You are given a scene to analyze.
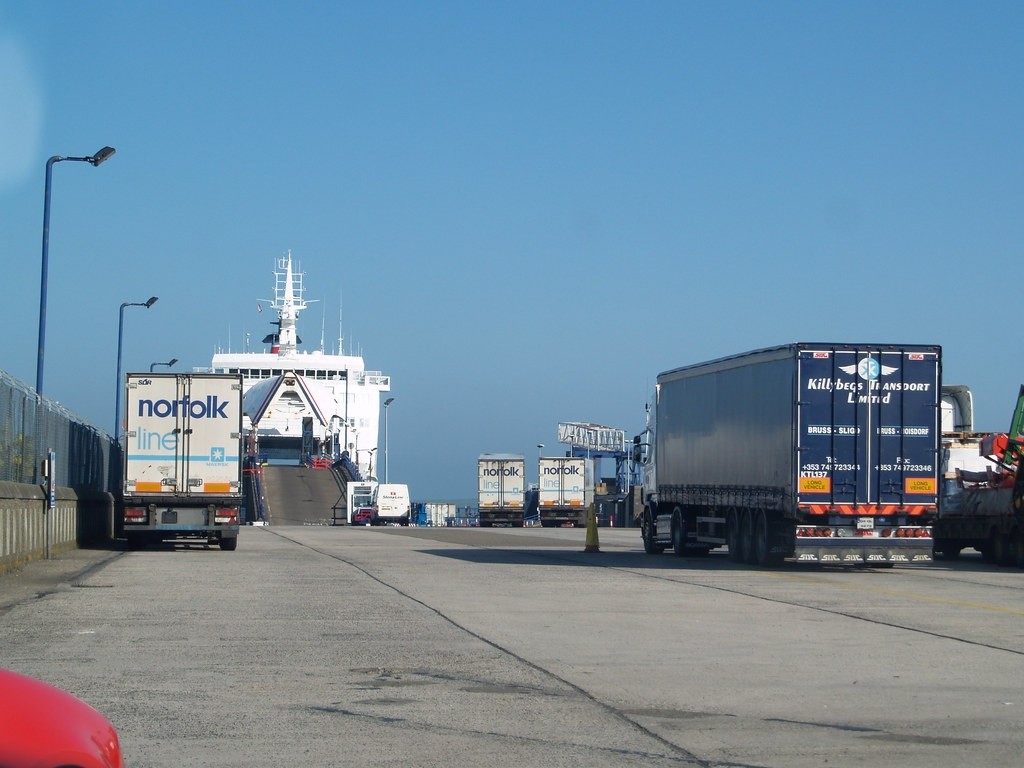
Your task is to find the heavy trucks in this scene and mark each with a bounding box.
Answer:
[933,384,1024,567]
[632,341,942,566]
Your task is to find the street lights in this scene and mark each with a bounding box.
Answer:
[150,358,178,372]
[114,296,159,444]
[384,397,394,483]
[35,146,116,485]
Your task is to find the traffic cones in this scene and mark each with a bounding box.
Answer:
[584,502,600,553]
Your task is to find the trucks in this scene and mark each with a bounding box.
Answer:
[477,452,525,527]
[373,483,411,526]
[538,457,594,527]
[347,482,379,525]
[122,372,244,550]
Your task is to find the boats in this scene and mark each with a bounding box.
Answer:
[210,248,392,482]
